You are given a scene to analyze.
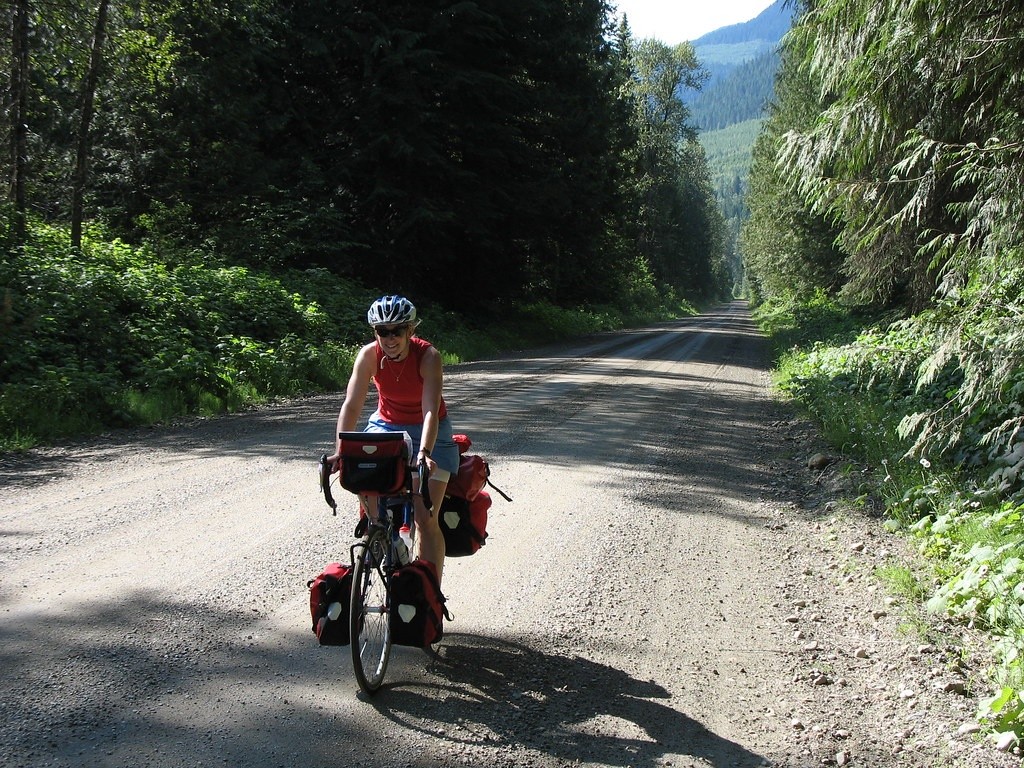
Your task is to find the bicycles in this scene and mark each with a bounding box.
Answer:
[318,433,472,698]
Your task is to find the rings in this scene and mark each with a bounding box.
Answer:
[432,473,435,475]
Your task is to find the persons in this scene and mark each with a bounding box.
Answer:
[583,299,592,314]
[319,296,460,592]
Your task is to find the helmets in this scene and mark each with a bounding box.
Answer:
[368,294,416,327]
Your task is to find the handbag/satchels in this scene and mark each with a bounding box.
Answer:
[446,455,490,502]
[439,491,494,558]
[338,430,412,498]
[388,559,454,647]
[309,562,364,647]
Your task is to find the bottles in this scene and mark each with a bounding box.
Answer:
[400,523,412,563]
[393,531,408,565]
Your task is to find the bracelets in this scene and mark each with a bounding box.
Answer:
[419,446,430,457]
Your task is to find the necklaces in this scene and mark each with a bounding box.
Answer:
[385,354,408,382]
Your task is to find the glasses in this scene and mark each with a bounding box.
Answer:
[375,324,408,338]
